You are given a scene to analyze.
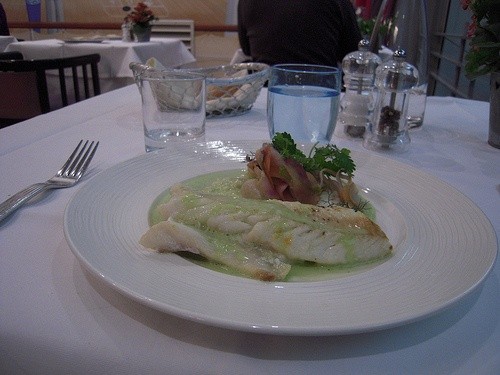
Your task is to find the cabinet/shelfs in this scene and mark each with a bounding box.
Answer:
[151,19,194,56]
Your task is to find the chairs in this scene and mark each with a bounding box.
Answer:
[0,52,101,119]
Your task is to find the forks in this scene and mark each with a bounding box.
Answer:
[0,139,100,221]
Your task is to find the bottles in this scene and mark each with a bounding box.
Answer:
[121,25,130,41]
[372,0,428,129]
[364,49,420,155]
[340,39,382,138]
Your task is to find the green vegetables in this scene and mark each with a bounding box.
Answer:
[242,132,356,177]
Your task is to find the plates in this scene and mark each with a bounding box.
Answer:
[62,137,498,335]
[61,39,103,43]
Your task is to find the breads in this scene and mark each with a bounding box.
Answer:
[193,69,248,100]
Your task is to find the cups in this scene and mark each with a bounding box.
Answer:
[135,70,207,152]
[267,64,342,146]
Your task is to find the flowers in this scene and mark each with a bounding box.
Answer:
[129,2,158,25]
[462,0,500,80]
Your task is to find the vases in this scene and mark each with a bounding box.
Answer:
[488,70,500,149]
[134,25,152,42]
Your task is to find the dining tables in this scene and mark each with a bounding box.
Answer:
[0,82,500,375]
[4,38,196,115]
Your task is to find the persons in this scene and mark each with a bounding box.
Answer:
[237,0,360,92]
